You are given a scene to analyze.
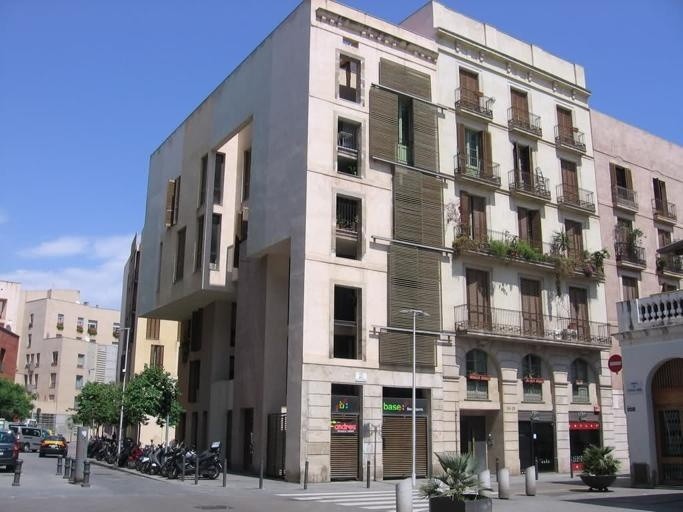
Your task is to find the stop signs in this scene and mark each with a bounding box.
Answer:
[608,355,623,373]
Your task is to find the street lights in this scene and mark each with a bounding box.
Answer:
[116,328,130,467]
[399,307,430,487]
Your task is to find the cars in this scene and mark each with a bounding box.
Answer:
[0,418,70,473]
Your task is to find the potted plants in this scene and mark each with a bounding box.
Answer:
[578,441,617,492]
[419,451,495,512]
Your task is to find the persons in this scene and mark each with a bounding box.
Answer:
[112,431,116,440]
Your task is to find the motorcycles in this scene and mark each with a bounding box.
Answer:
[87,432,223,479]
